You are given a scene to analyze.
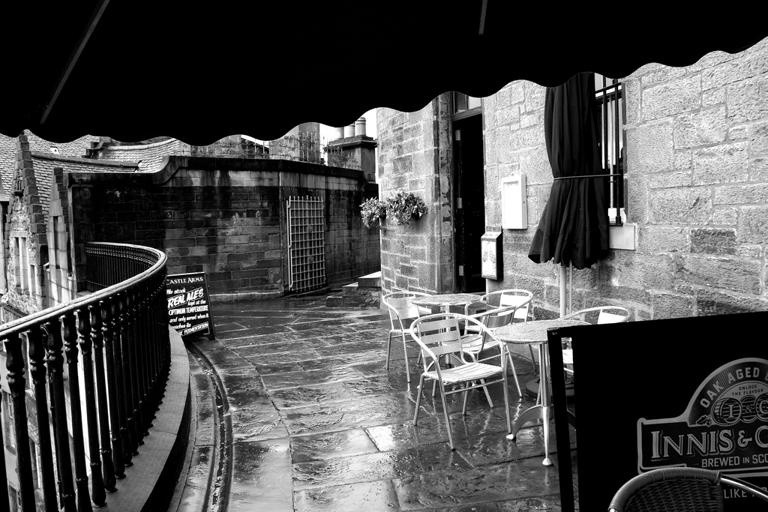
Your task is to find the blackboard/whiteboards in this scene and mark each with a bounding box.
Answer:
[166,271,213,336]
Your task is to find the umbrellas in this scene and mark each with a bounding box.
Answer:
[528,70,610,317]
[0,0,768,145]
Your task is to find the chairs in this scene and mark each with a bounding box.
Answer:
[381,285,633,452]
[607,463,768,512]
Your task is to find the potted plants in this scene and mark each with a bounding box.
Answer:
[357,187,428,231]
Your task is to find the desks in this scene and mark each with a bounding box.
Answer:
[497,316,592,468]
